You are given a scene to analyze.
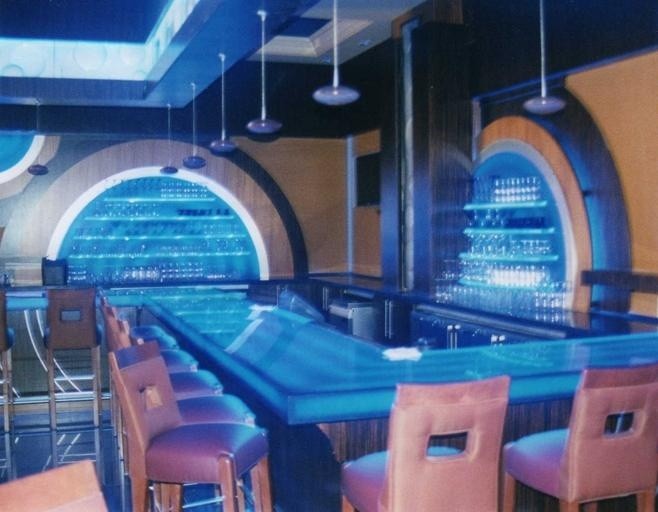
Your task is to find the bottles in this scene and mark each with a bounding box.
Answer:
[432,174,568,315]
[64,176,249,286]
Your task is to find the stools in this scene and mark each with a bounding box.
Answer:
[40,284,104,430]
[338,378,509,509]
[0,287,21,435]
[98,294,275,512]
[503,368,658,509]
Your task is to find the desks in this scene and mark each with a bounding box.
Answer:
[292,268,657,346]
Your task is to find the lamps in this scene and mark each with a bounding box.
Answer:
[244,12,285,145]
[520,1,565,121]
[182,80,207,173]
[161,102,178,174]
[207,50,240,158]
[28,98,49,176]
[309,3,359,109]
